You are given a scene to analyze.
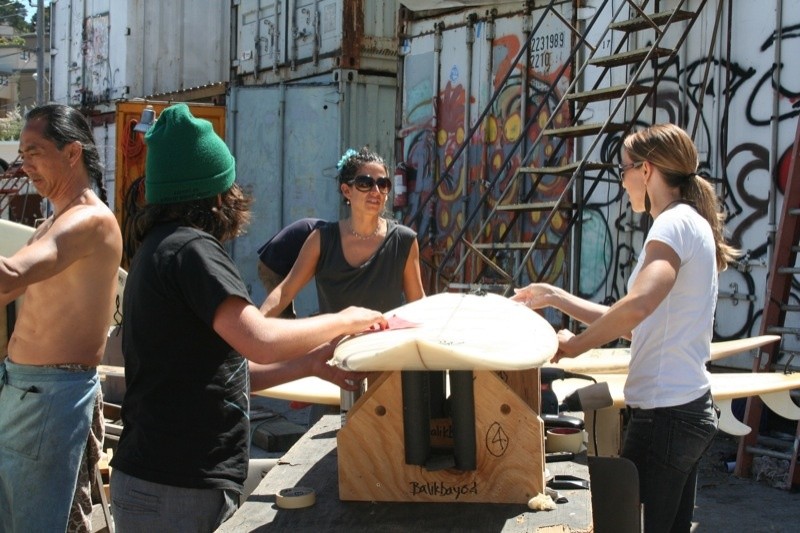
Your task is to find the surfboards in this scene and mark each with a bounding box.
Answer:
[333,291,560,372]
[0,219,129,325]
[249,333,800,407]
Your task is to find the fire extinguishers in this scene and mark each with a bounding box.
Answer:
[393,162,408,207]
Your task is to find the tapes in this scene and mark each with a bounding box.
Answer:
[275,487,316,509]
[547,428,583,453]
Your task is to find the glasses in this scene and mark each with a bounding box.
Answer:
[346,174,392,194]
[617,160,644,181]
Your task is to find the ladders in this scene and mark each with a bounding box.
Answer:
[734,119,800,491]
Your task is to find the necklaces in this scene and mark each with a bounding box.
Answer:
[348,215,382,241]
[660,198,682,214]
[47,188,88,226]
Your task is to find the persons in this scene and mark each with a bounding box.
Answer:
[106,105,390,533]
[509,124,743,533]
[257,218,329,319]
[257,148,428,428]
[0,105,123,533]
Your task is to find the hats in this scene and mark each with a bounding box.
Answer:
[144,104,237,201]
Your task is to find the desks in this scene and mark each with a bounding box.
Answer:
[215,415,595,533]
[546,334,780,370]
[248,373,800,455]
